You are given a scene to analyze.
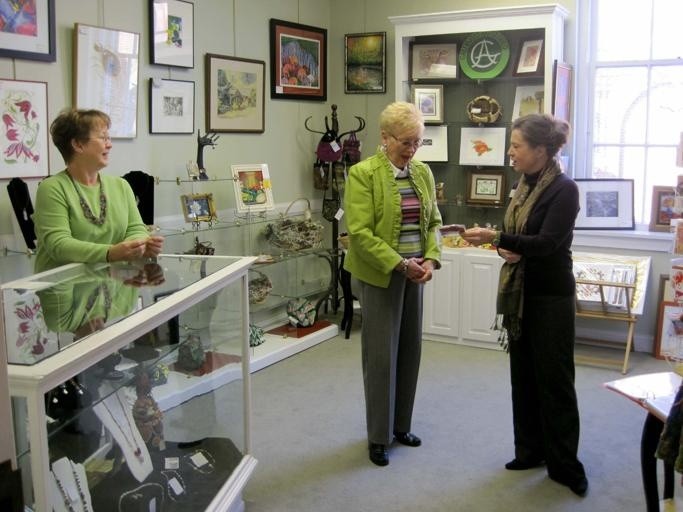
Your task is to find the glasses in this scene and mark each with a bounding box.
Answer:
[77,130,112,141]
[385,128,422,148]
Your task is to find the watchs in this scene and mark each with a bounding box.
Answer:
[395,258,409,274]
[492,231,502,249]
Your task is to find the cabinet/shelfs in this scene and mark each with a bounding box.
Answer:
[3,250,260,511]
[45,178,339,412]
[380,5,572,352]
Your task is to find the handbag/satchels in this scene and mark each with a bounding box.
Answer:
[343,131,361,164]
[266,198,322,256]
[196,242,214,256]
[178,339,202,369]
[318,130,341,161]
[285,298,319,326]
[322,167,340,221]
[313,157,330,189]
[249,268,272,304]
[252,326,262,346]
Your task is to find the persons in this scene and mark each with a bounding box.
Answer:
[34,260,164,341]
[460,112,589,497]
[32,108,164,271]
[346,100,443,465]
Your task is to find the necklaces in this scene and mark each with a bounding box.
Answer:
[119,482,165,512]
[160,468,187,505]
[66,168,107,225]
[182,450,217,476]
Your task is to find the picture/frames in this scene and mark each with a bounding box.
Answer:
[648,184,680,234]
[268,17,328,105]
[0,79,50,181]
[150,0,194,70]
[72,23,139,140]
[404,82,443,121]
[204,53,266,136]
[656,299,683,361]
[406,40,460,82]
[179,193,218,225]
[570,178,635,232]
[511,84,544,122]
[148,76,196,137]
[463,174,506,206]
[0,1,57,65]
[654,274,683,356]
[229,164,276,215]
[344,31,386,96]
[513,36,542,74]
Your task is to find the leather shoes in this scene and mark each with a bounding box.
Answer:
[368,442,389,465]
[569,478,587,494]
[394,431,420,447]
[506,458,525,470]
